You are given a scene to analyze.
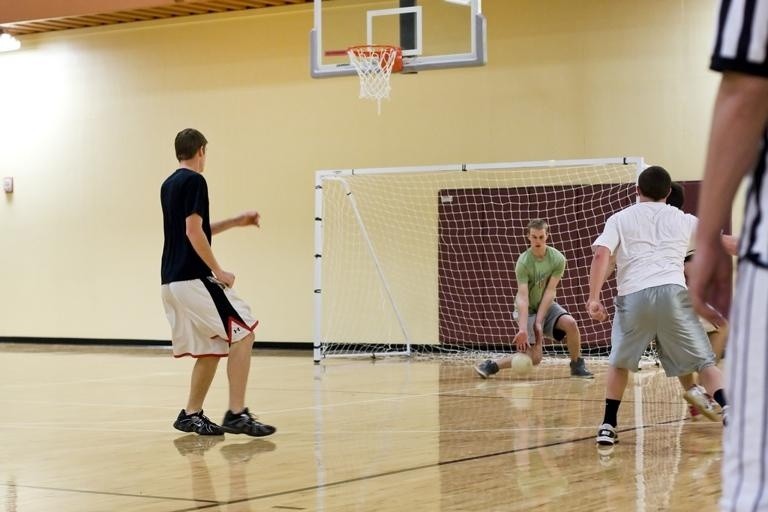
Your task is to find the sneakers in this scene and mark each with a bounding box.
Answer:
[174,409,224,435]
[595,423,619,445]
[221,407,275,436]
[570,357,594,379]
[685,384,730,426]
[474,360,499,379]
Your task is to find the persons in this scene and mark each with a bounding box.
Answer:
[509,380,572,511]
[652,182,730,424]
[156,128,275,438]
[583,164,730,448]
[170,433,276,512]
[684,0,768,509]
[593,439,690,512]
[474,217,597,380]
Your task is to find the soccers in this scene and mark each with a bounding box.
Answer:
[511,354,533,376]
